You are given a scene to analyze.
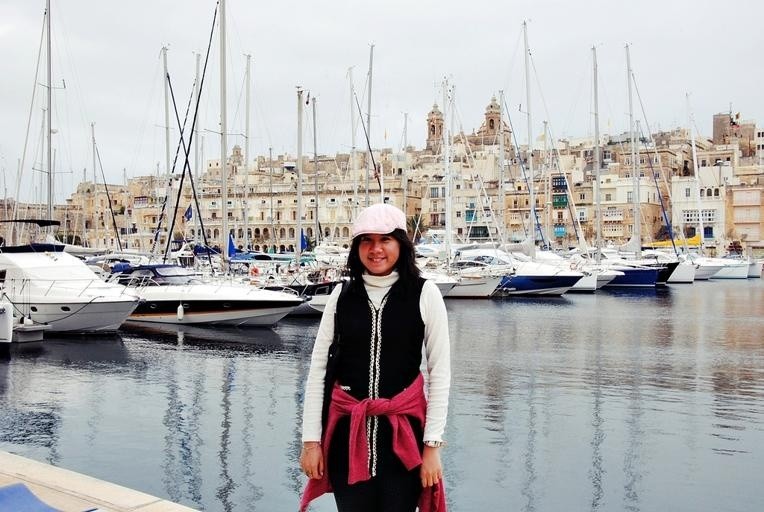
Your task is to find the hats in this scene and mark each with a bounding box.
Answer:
[347,200,409,242]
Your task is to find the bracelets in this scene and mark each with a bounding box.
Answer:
[303,444,320,450]
[423,441,443,448]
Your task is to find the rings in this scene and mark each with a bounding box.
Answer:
[305,471,311,477]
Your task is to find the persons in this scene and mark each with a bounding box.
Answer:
[300,204,451,512]
